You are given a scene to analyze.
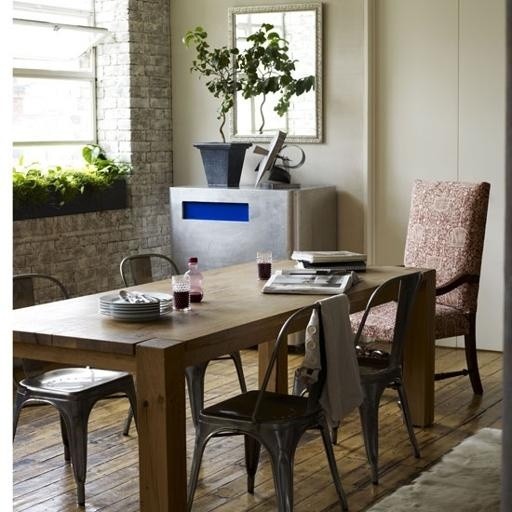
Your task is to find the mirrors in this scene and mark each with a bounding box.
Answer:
[228,2,323,145]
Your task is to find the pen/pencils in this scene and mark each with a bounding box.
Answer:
[290,269,347,275]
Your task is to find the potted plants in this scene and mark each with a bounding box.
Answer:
[182,26,245,187]
[14,142,127,218]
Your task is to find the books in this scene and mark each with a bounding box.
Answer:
[253,247,369,296]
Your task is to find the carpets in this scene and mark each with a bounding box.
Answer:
[365,429,501,510]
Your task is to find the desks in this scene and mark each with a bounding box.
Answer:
[11,259,437,512]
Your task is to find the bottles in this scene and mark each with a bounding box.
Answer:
[184,254,203,303]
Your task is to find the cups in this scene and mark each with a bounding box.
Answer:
[257,250,272,281]
[171,273,190,313]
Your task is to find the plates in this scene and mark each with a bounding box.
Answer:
[98,290,174,324]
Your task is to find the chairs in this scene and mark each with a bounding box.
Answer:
[11,272,138,506]
[349,179,490,410]
[290,271,423,484]
[119,253,248,443]
[185,292,350,512]
